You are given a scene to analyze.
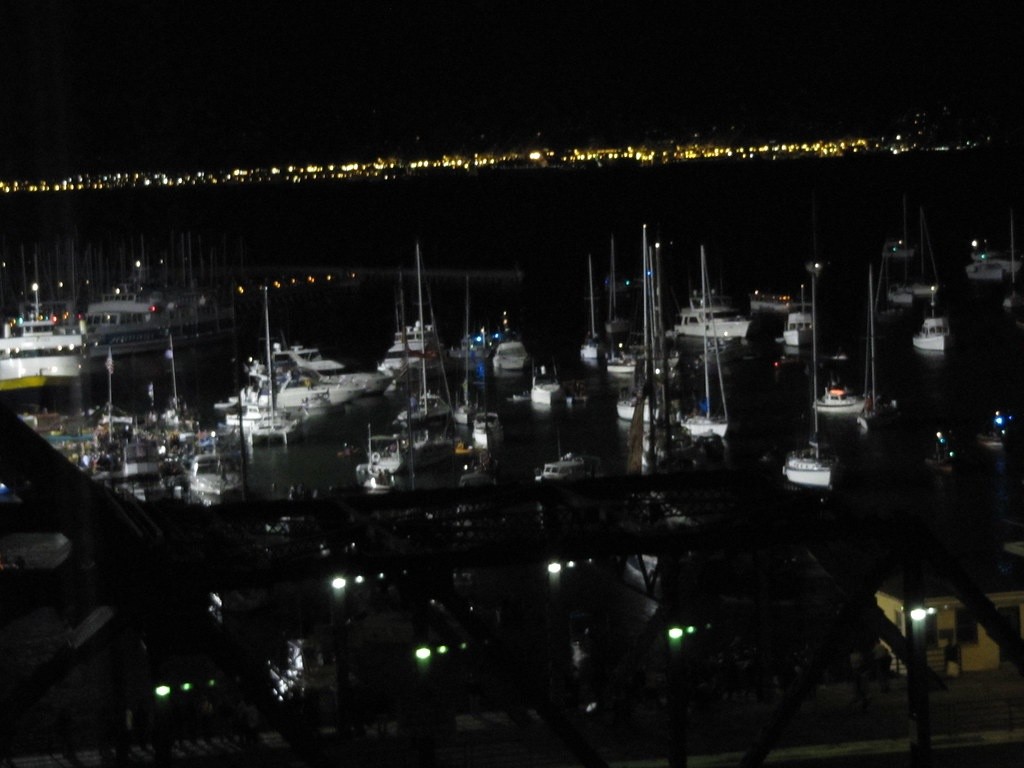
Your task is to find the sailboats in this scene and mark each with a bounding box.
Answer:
[0,205,1024,507]
